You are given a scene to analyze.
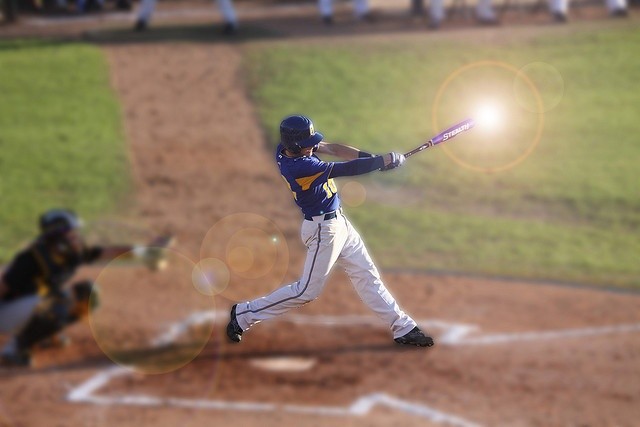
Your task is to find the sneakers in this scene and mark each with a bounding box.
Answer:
[394,326,434,348]
[227,304,243,342]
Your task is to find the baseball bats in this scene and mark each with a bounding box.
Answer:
[404,116,475,158]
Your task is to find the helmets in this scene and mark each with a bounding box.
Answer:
[40,210,83,274]
[280,115,324,154]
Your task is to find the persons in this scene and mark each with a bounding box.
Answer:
[226,114,434,347]
[426,1,501,27]
[315,1,376,21]
[131,2,243,32]
[549,1,632,20]
[1,207,149,365]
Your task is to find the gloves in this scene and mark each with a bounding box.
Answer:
[389,152,406,167]
[375,155,396,170]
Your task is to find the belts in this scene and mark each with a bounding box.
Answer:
[305,205,342,221]
